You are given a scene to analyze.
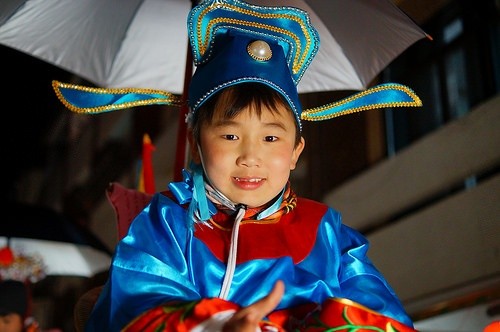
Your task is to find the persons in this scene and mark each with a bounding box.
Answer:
[51,0,422,332]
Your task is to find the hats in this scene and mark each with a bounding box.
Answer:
[52,0,422,133]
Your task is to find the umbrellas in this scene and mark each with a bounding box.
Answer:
[0,0,433,182]
